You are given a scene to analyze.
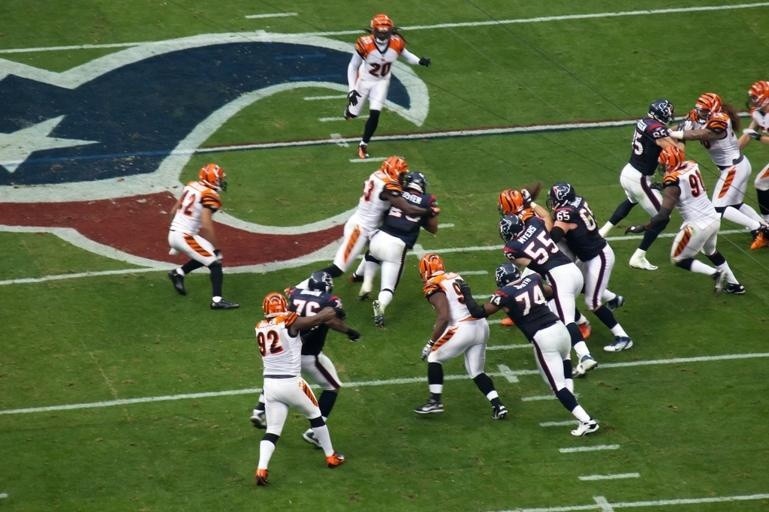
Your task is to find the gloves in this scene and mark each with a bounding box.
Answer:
[625,225,644,235]
[420,340,434,361]
[348,91,361,105]
[334,307,346,320]
[419,57,430,67]
[456,280,469,289]
[650,182,663,191]
[346,329,360,341]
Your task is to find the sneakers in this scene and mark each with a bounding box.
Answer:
[572,295,634,379]
[491,405,509,420]
[629,257,659,270]
[413,399,445,414]
[168,269,186,295]
[349,272,384,328]
[500,317,513,326]
[302,428,322,448]
[344,109,351,121]
[326,451,345,468]
[570,418,600,437]
[751,228,769,250]
[358,145,369,159]
[711,269,746,295]
[256,469,269,486]
[210,299,240,310]
[250,409,267,429]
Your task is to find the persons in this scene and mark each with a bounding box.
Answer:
[167,163,239,310]
[598,82,769,294]
[284,156,439,329]
[415,253,508,419]
[456,181,634,435]
[342,14,431,158]
[249,272,360,486]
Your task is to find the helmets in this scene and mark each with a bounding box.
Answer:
[382,155,426,195]
[647,80,769,125]
[199,163,227,192]
[371,14,394,44]
[419,253,445,283]
[309,271,335,293]
[262,292,289,319]
[494,262,522,286]
[656,145,686,175]
[495,182,524,243]
[547,183,576,210]
